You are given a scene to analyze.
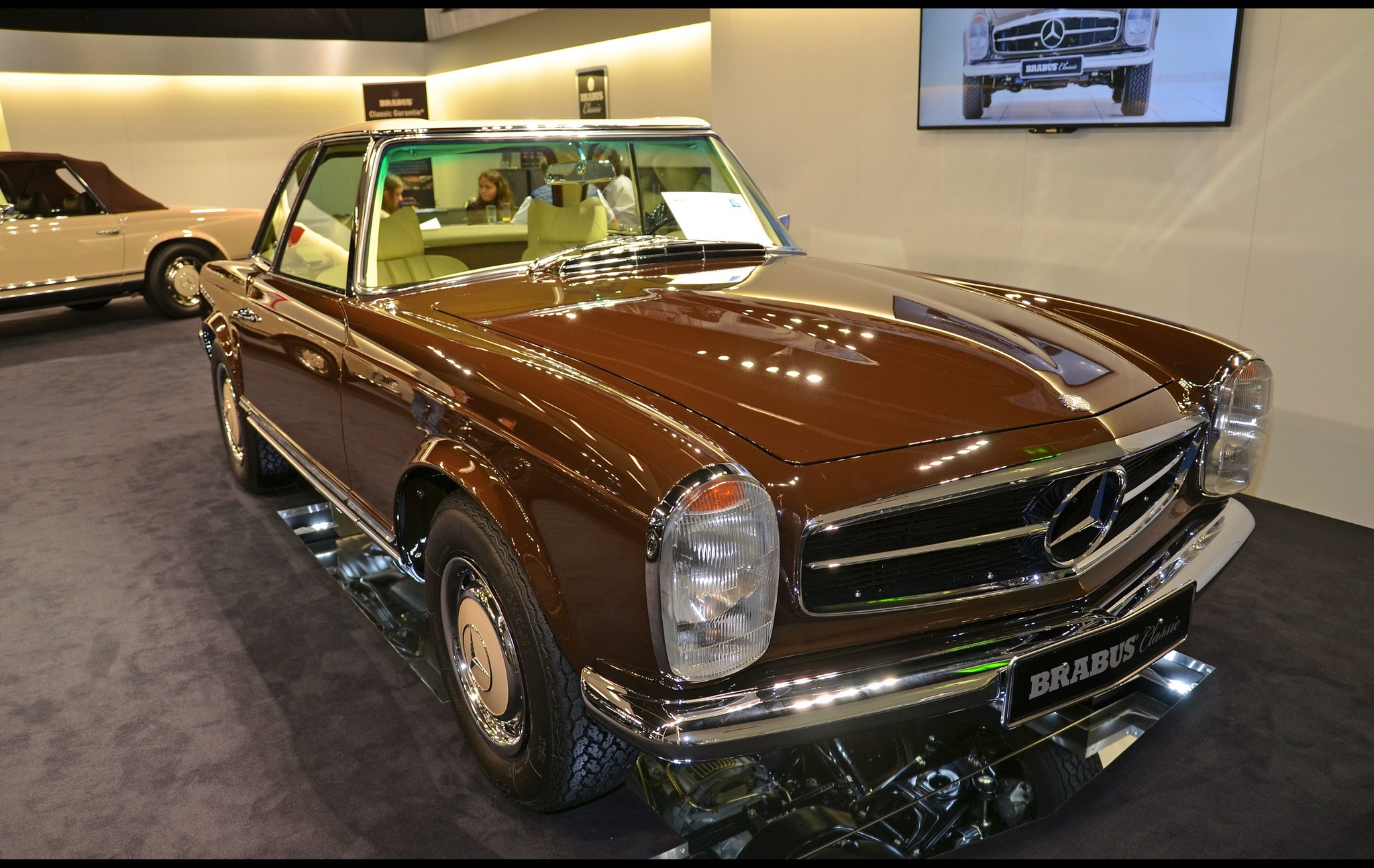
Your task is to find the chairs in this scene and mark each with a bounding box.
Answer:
[377,206,469,287]
[62,192,95,215]
[17,194,50,217]
[521,196,607,263]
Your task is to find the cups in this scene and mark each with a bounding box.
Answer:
[501,202,511,222]
[486,205,497,224]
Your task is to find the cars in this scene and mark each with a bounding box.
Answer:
[200,114,1272,858]
[0,151,268,320]
[963,9,1161,120]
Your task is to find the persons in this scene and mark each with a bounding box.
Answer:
[591,144,644,231]
[467,169,516,224]
[508,153,618,230]
[339,171,404,233]
